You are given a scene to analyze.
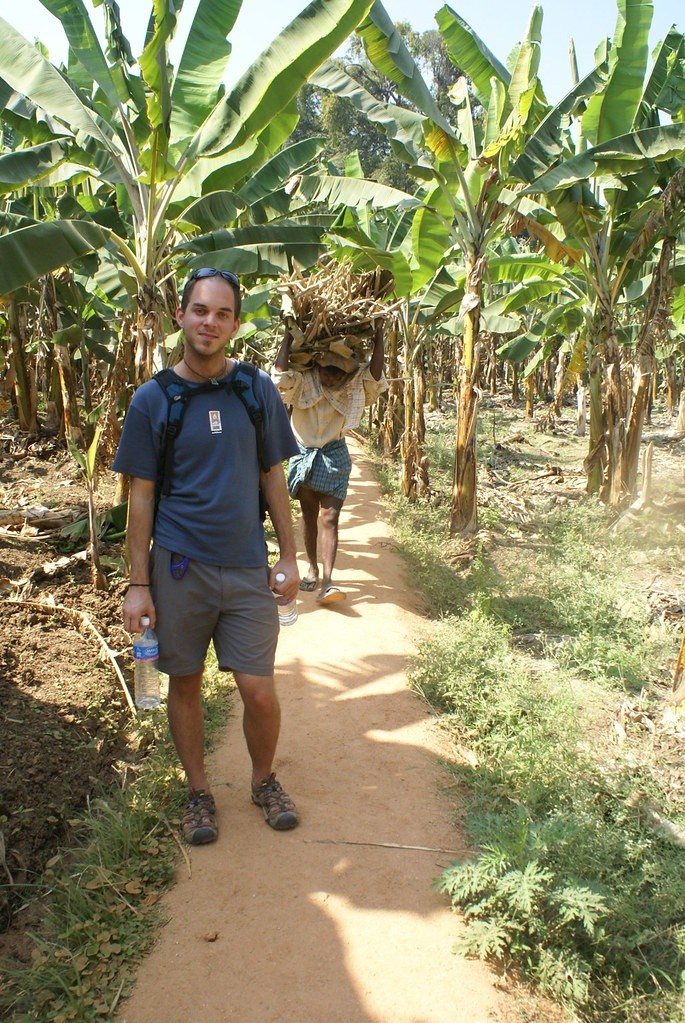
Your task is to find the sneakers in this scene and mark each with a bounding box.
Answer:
[180,786,218,844]
[251,772,300,831]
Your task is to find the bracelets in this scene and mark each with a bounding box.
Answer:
[128,583,150,587]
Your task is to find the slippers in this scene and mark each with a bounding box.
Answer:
[298,575,319,591]
[316,585,346,605]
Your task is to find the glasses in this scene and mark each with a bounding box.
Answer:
[191,267,240,290]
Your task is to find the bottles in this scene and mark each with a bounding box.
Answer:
[133,616,161,709]
[272,573,298,626]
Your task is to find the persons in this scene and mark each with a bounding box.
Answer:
[109,268,301,845]
[270,309,391,605]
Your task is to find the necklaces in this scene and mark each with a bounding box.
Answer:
[183,357,227,385]
